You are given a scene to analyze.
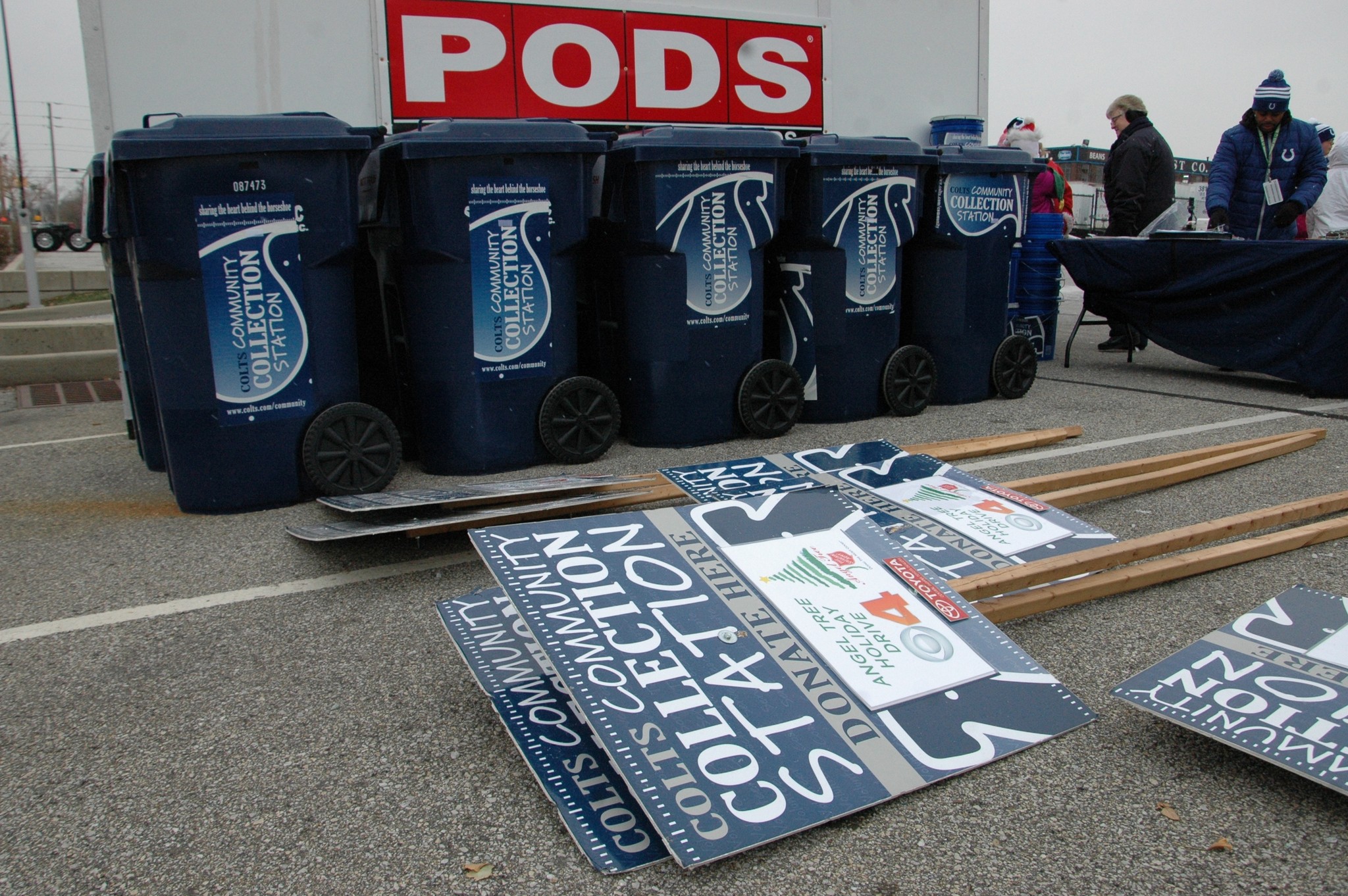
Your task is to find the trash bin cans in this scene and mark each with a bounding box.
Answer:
[84,110,1038,514]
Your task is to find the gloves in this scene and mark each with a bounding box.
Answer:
[1270,202,1302,226]
[1207,209,1230,232]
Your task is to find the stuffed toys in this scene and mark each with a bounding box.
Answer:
[996,115,1074,236]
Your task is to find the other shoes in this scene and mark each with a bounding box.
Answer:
[1098,330,1146,353]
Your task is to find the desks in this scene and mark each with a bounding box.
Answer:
[1058,228,1348,401]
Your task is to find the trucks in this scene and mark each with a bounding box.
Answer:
[1067,180,1210,239]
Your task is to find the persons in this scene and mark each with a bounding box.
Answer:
[1204,68,1330,241]
[1006,126,1065,214]
[1097,94,1176,352]
[1304,131,1348,239]
[1292,118,1335,240]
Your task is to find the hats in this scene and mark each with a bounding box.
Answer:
[1309,118,1335,144]
[1252,70,1291,113]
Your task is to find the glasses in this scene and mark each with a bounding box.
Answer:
[1110,114,1122,125]
